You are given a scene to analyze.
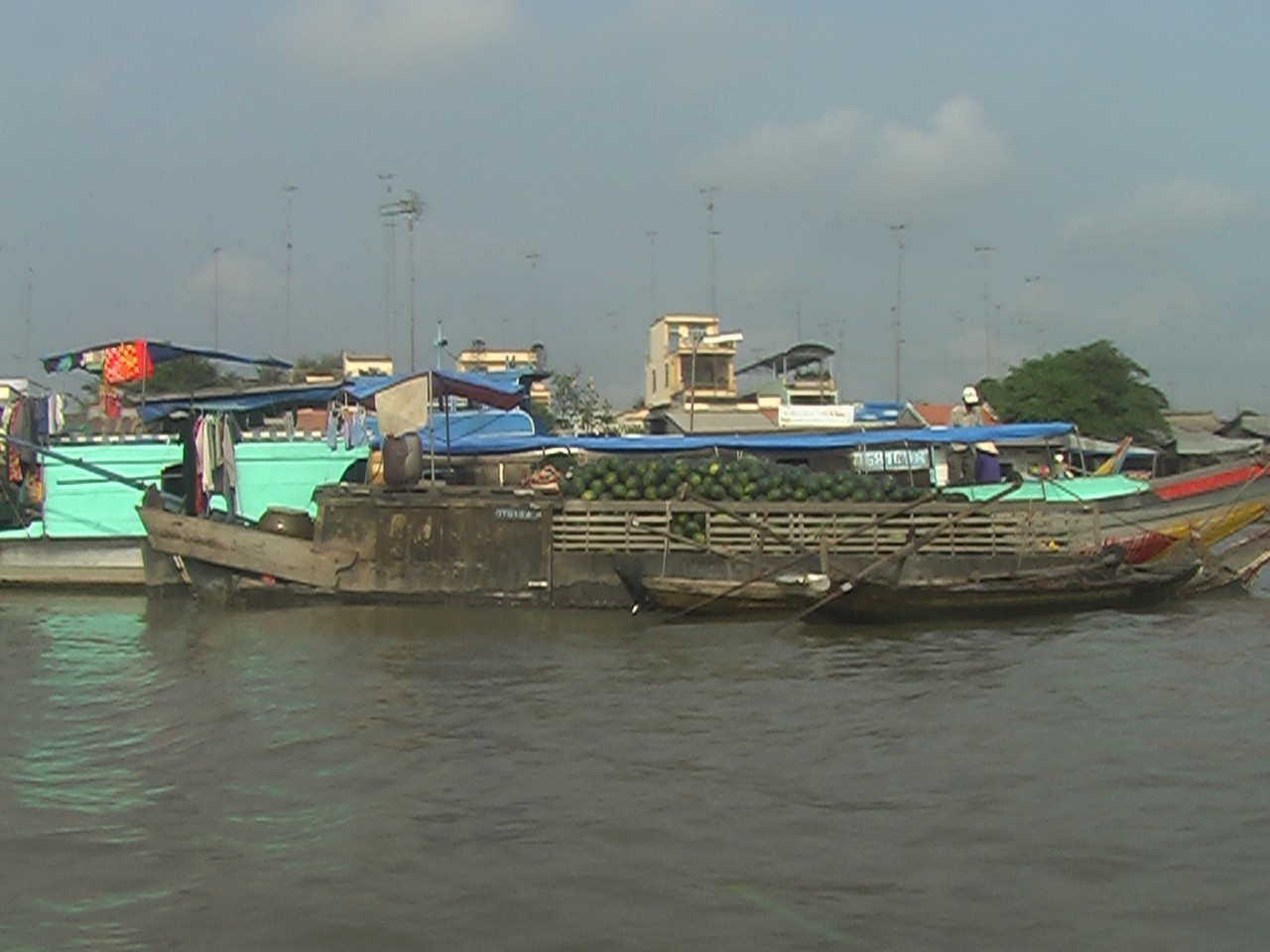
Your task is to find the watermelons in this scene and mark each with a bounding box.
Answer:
[569,453,967,553]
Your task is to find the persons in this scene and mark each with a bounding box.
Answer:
[947,386,979,485]
[975,441,1000,483]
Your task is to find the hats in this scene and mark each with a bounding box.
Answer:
[963,387,978,404]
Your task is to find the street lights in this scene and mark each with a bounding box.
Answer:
[381,189,422,374]
[890,224,905,419]
[213,246,223,350]
[286,184,295,361]
[975,245,992,375]
[380,171,394,359]
[526,251,541,347]
[701,185,722,314]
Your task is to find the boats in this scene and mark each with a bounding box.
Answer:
[0,340,1270,624]
